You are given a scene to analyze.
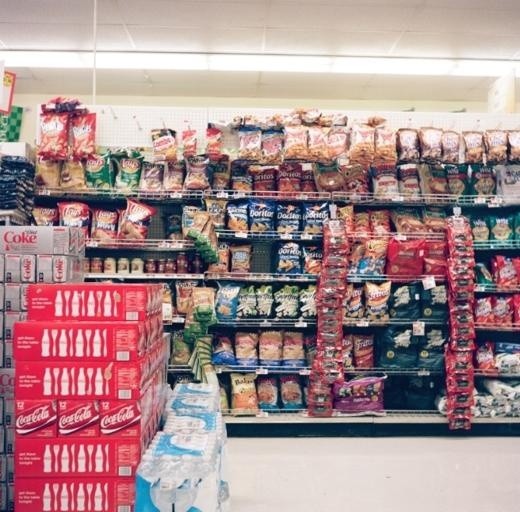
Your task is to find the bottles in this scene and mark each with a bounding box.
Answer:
[99,402,144,436]
[41,328,50,357]
[43,483,51,511]
[57,402,100,434]
[72,291,79,317]
[43,445,52,473]
[61,368,70,395]
[61,445,69,471]
[94,483,102,511]
[95,368,103,395]
[61,483,68,511]
[16,405,58,433]
[78,444,86,472]
[78,368,86,396]
[75,328,84,357]
[88,291,95,316]
[77,483,84,511]
[59,328,68,357]
[43,368,52,395]
[93,328,102,357]
[54,291,63,316]
[104,291,112,317]
[95,445,103,472]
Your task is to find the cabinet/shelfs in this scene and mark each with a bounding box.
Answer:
[32,186,520,438]
